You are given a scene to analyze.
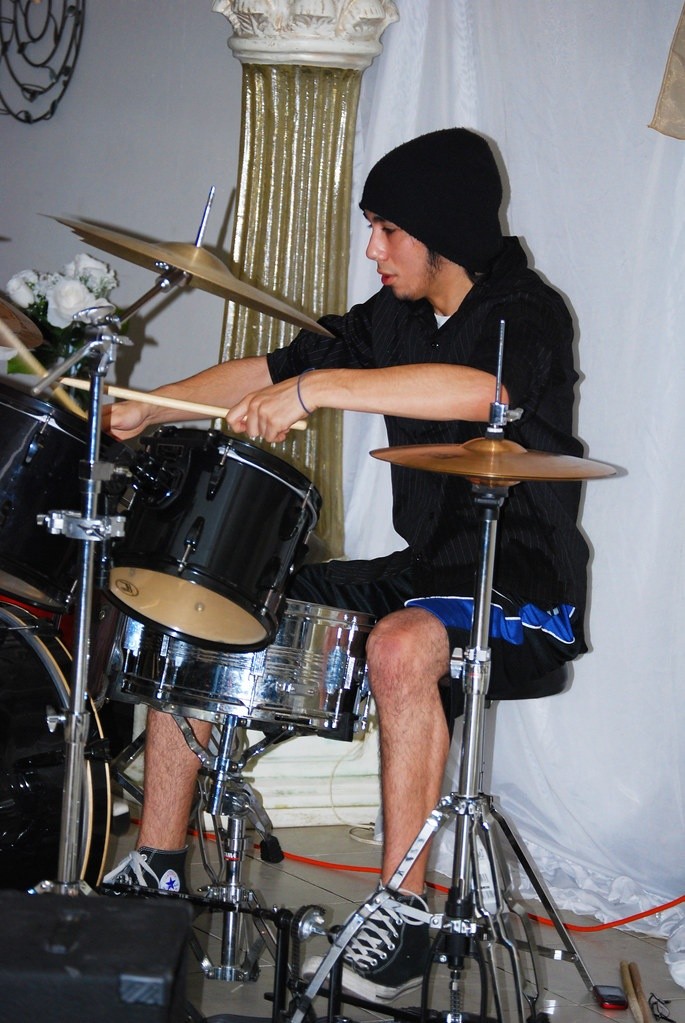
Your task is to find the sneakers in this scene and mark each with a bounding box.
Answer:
[302,894,440,1005]
[94,850,191,899]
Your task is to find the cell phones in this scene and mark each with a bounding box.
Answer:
[593,985,628,1009]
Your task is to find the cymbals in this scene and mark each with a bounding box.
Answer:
[0,295,45,349]
[34,211,337,341]
[368,437,619,487]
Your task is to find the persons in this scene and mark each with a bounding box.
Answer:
[101,128,592,1004]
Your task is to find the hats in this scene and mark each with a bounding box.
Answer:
[360,126,507,268]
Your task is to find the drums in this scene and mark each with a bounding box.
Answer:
[123,594,379,745]
[100,424,324,655]
[0,600,112,899]
[0,383,135,615]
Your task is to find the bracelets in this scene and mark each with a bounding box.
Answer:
[296,367,315,415]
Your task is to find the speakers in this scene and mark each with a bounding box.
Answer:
[0,890,205,1023]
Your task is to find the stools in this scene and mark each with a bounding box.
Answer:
[345,637,575,960]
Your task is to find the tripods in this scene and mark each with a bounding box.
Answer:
[195,484,595,1023]
